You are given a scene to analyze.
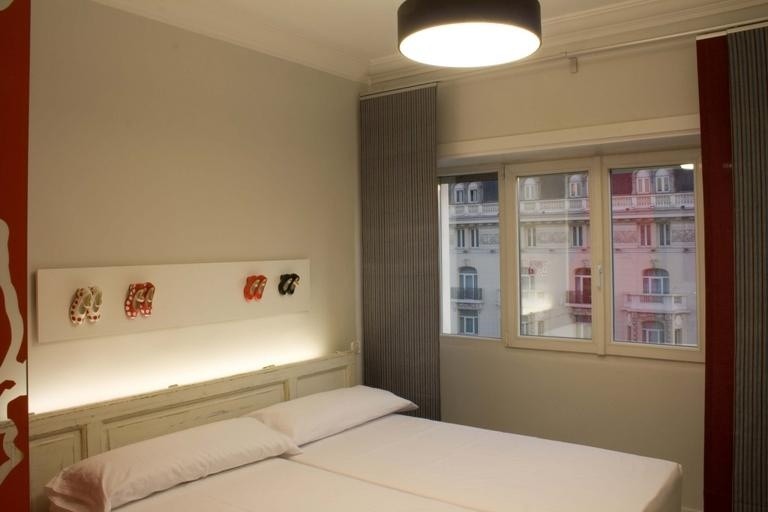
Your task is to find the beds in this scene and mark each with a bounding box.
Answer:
[29,351,683,512]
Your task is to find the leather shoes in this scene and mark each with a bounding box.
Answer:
[69,287,93,326]
[244,275,260,302]
[254,274,267,301]
[140,282,156,319]
[287,273,301,295]
[125,282,145,320]
[86,285,104,323]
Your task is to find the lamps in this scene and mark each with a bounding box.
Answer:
[397,0,541,69]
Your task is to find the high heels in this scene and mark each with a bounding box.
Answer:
[278,273,293,296]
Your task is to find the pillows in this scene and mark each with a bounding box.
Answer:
[242,385,419,446]
[45,416,302,512]
